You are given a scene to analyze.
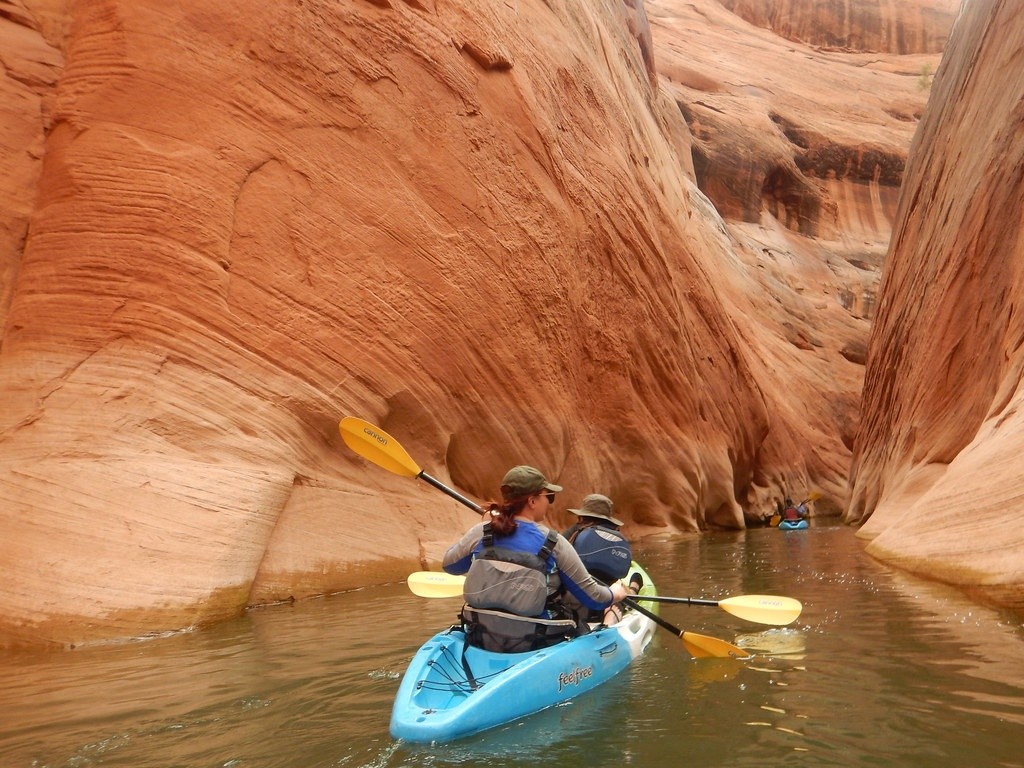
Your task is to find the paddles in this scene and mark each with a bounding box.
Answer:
[337,413,753,662]
[405,569,804,627]
[770,490,823,527]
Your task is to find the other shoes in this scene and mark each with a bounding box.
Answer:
[629,573,643,595]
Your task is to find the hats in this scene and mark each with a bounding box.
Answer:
[501,466,563,499]
[566,494,624,527]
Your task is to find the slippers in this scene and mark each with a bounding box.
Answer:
[602,603,623,623]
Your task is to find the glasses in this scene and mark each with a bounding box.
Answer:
[533,490,555,504]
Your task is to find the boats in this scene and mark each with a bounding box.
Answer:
[779,520,809,530]
[388,559,660,743]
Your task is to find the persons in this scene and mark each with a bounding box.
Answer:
[549,494,643,621]
[772,496,806,522]
[442,465,628,653]
